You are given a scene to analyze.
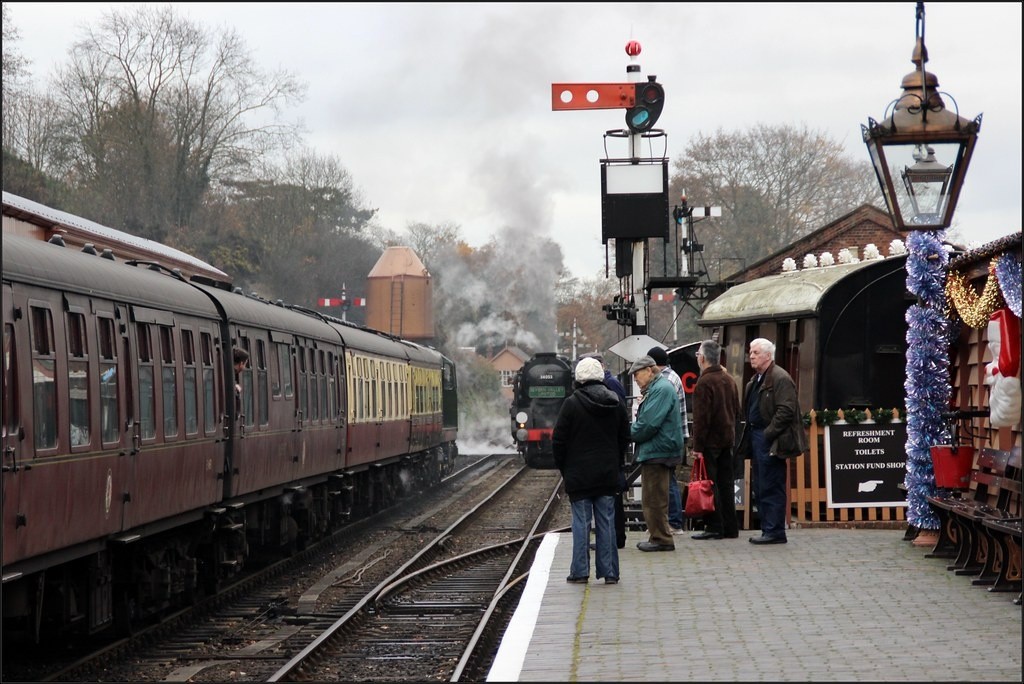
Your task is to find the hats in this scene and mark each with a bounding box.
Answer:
[628,355,656,375]
[580,352,603,363]
[574,357,605,384]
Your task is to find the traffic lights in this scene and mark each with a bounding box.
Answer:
[625,74,665,133]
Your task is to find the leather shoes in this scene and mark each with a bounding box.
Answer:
[567,575,589,583]
[723,532,738,538]
[691,530,723,540]
[605,576,618,584]
[749,536,786,544]
[590,544,597,550]
[636,542,675,551]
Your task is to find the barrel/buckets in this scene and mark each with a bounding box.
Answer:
[930,424,974,490]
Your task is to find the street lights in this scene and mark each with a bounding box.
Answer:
[860,1,984,548]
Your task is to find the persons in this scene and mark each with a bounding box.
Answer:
[692,339,742,539]
[735,337,808,546]
[550,358,632,584]
[233,346,249,395]
[579,352,628,551]
[629,355,684,552]
[636,346,690,535]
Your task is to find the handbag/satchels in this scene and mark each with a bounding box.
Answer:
[684,456,715,517]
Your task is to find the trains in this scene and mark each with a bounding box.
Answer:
[0,189,459,655]
[509,352,580,471]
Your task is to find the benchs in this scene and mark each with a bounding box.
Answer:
[924,447,1010,570]
[954,446,1024,585]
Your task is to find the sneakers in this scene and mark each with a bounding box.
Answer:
[647,524,683,536]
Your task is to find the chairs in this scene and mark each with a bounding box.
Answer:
[982,517,1023,606]
